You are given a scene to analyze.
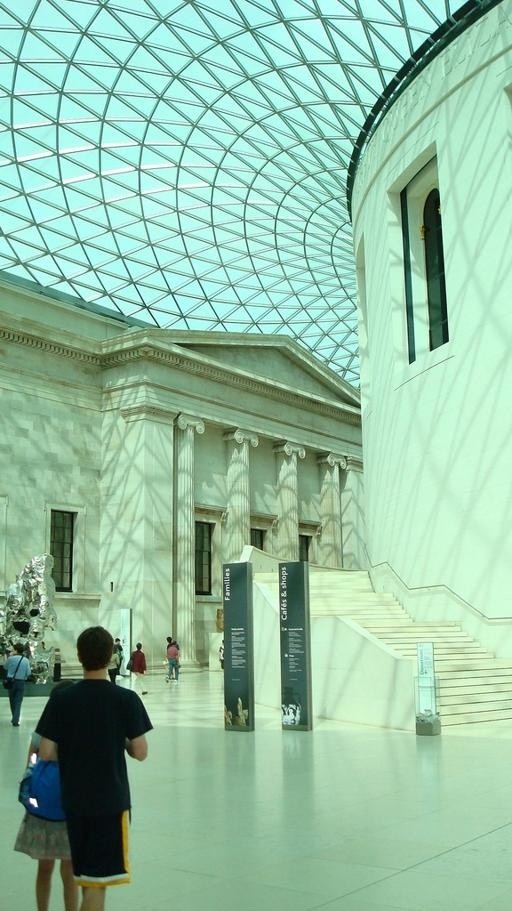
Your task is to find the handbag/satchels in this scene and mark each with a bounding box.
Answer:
[126,659,133,671]
[3,677,15,689]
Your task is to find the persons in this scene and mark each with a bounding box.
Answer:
[3,643,32,726]
[12,679,80,911]
[130,642,149,695]
[166,637,180,681]
[108,637,123,686]
[33,625,153,911]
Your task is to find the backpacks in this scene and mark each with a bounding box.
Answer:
[17,759,69,823]
